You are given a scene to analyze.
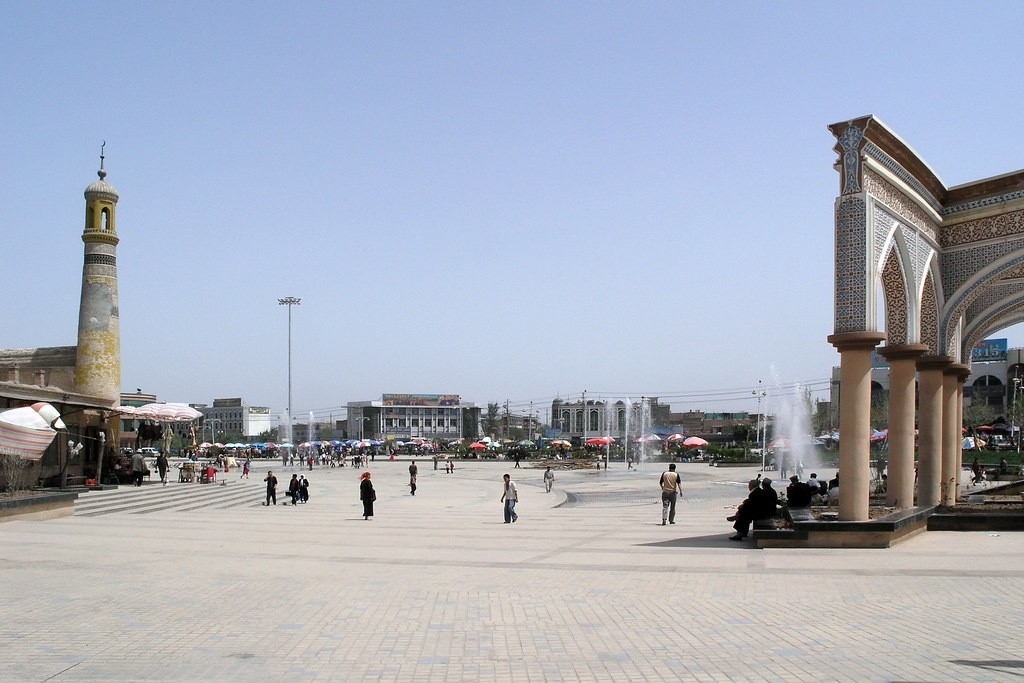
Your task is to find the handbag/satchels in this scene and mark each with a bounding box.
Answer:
[286,490,292,496]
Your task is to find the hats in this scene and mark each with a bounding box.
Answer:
[763,478,772,485]
[137,449,143,452]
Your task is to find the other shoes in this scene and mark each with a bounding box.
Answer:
[513,516,518,522]
[727,516,736,521]
[729,535,742,541]
[504,522,510,523]
[662,521,666,525]
[670,522,675,524]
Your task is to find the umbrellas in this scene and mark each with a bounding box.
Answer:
[636,432,662,444]
[976,424,992,431]
[354,441,370,449]
[329,440,346,448]
[199,441,213,447]
[280,443,294,449]
[211,442,225,449]
[517,438,536,448]
[225,442,235,448]
[668,434,686,444]
[469,442,487,451]
[769,436,792,449]
[1006,425,1020,432]
[369,440,381,446]
[550,439,572,449]
[683,437,709,448]
[234,443,247,448]
[992,423,1008,431]
[299,441,312,448]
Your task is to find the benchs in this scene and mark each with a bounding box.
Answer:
[54,476,86,486]
[108,474,120,485]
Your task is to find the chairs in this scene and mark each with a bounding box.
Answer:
[142,470,150,482]
[183,463,216,484]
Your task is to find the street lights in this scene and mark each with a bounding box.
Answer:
[218,430,223,443]
[202,426,211,443]
[522,409,540,441]
[460,406,470,438]
[356,410,370,439]
[752,380,767,448]
[277,296,302,444]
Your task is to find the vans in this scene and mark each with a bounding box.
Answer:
[142,448,159,453]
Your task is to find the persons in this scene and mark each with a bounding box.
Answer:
[130,431,1024,541]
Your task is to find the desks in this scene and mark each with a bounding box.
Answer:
[179,468,191,482]
[201,470,217,484]
